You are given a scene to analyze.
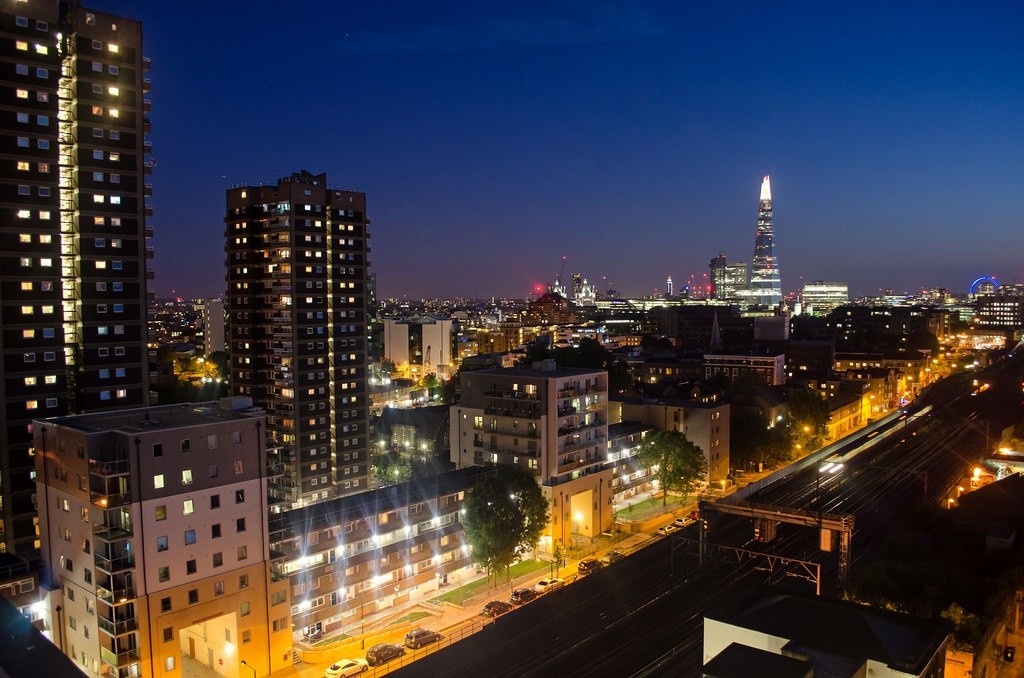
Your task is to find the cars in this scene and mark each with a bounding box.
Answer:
[689,511,699,520]
[482,601,513,618]
[324,658,370,678]
[510,587,539,605]
[657,524,677,536]
[535,577,565,594]
[675,517,693,527]
[602,551,624,564]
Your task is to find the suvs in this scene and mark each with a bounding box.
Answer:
[366,643,404,666]
[578,558,603,574]
[404,628,442,649]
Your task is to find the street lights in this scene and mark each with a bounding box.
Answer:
[436,571,441,605]
[393,586,400,625]
[957,486,964,499]
[947,498,955,510]
[720,480,726,495]
[360,579,381,650]
[575,512,583,554]
[796,445,801,461]
[241,660,256,678]
